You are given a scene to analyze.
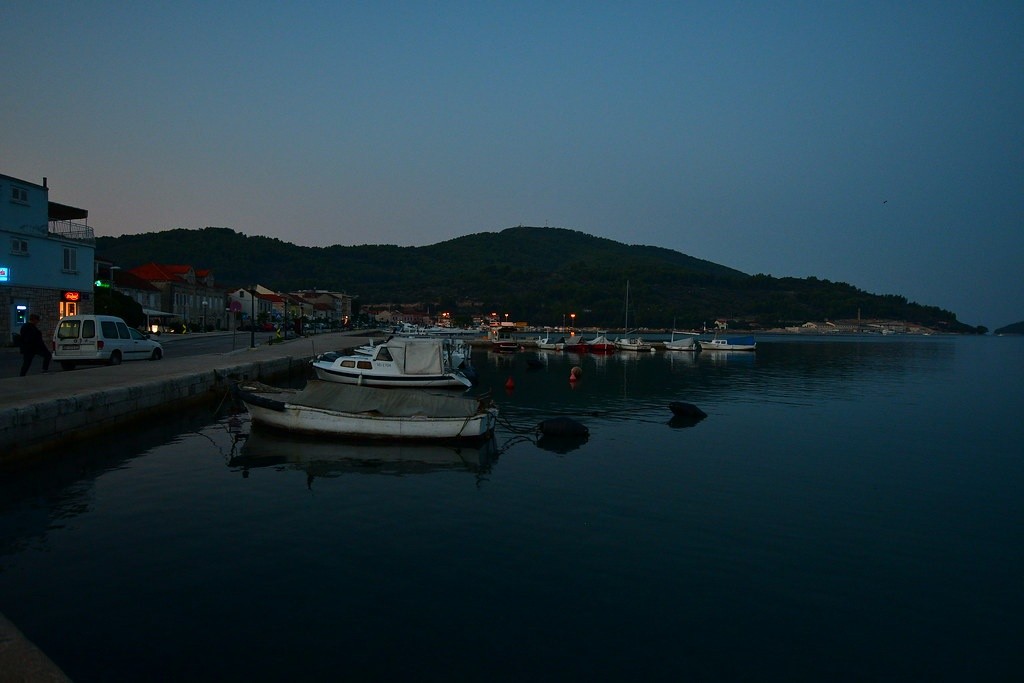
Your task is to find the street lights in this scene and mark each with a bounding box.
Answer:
[312,307,317,335]
[202,301,210,333]
[299,300,305,337]
[280,294,288,340]
[248,282,258,350]
[225,307,230,331]
[330,312,334,333]
[108,266,121,298]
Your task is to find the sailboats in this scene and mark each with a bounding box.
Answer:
[614,279,651,351]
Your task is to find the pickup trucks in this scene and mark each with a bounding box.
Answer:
[50,314,165,371]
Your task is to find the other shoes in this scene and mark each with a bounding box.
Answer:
[42,370,55,375]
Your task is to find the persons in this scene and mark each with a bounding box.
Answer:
[274,324,280,339]
[183,319,192,335]
[20,313,52,378]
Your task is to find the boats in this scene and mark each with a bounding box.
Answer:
[576,331,616,351]
[384,315,481,337]
[536,328,575,352]
[490,325,520,350]
[663,330,702,353]
[207,368,500,442]
[696,331,758,351]
[308,335,475,389]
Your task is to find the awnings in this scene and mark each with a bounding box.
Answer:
[142,310,182,316]
